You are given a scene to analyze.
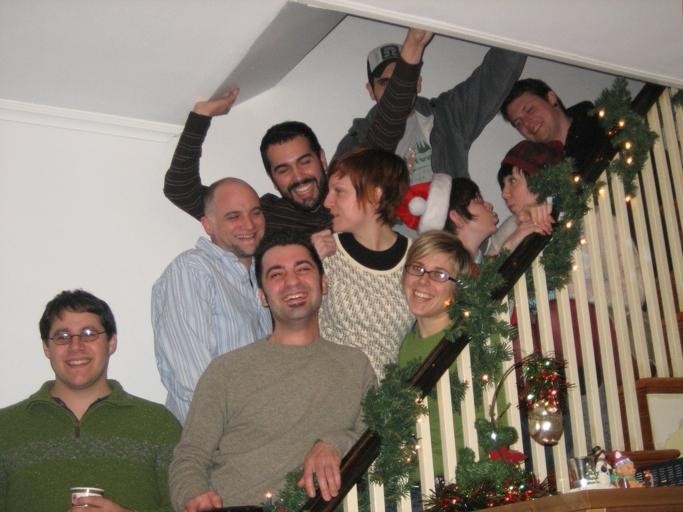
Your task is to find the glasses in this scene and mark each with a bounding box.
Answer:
[41,326,110,344]
[403,261,462,285]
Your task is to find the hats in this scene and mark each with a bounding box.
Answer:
[393,169,453,234]
[500,138,565,177]
[366,43,405,77]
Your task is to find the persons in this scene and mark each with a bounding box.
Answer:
[307,146,415,390]
[485,137,657,408]
[395,231,483,509]
[0,289,183,512]
[151,177,271,426]
[396,174,498,266]
[499,76,619,180]
[169,226,380,510]
[164,26,438,235]
[330,41,528,182]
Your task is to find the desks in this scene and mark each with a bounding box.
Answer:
[471,483,682,512]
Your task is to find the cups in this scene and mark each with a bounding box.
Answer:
[70,485,105,510]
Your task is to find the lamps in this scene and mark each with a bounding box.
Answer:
[471,348,565,455]
